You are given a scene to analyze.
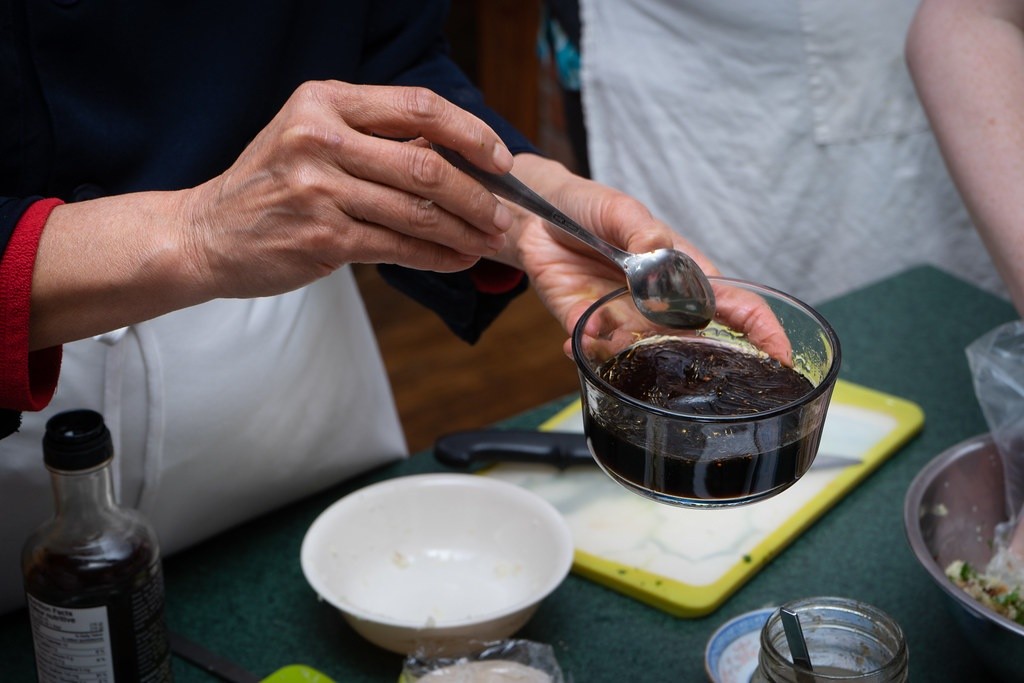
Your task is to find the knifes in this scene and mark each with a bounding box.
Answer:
[432,426,860,477]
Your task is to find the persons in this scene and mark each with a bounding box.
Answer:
[2,0,792,620]
[572,0,1024,331]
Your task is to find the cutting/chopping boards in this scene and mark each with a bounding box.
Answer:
[481,365,918,616]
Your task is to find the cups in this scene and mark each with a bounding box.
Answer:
[749,594,910,682]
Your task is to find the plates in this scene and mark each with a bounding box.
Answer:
[703,605,781,683]
[21,415,169,683]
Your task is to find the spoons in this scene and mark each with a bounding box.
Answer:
[430,139,714,330]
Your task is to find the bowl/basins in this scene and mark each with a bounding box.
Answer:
[299,471,572,662]
[902,432,1024,644]
[571,275,840,508]
